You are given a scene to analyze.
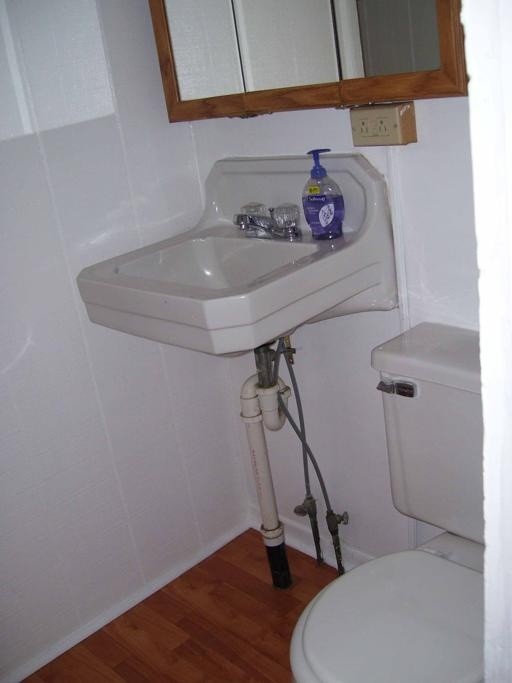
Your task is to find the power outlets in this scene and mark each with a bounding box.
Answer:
[350,101,416,147]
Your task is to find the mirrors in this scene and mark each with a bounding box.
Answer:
[149,0,469,122]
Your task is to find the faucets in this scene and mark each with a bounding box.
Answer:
[233,212,284,237]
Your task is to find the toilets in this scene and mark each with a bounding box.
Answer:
[289,324,484,682]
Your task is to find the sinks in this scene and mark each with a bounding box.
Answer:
[75,152,399,355]
